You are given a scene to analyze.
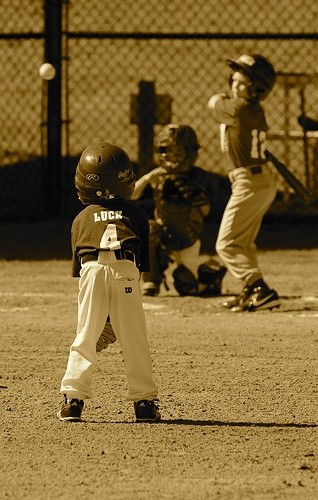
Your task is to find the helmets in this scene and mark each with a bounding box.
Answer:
[75,142,137,205]
[226,53,276,101]
[152,123,199,174]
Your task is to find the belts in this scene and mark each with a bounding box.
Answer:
[229,166,275,175]
[81,252,138,266]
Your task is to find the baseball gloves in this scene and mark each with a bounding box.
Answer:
[96,314,118,352]
[157,174,208,208]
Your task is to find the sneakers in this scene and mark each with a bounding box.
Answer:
[132,399,164,423]
[55,398,84,420]
[230,288,282,311]
[222,284,268,308]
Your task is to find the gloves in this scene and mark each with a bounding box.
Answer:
[172,263,198,295]
[194,262,228,296]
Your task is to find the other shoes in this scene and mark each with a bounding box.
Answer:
[140,274,169,298]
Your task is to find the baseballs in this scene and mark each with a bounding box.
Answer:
[39,62,56,79]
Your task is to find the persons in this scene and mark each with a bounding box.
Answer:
[56,142,161,423]
[208,53,281,313]
[130,124,233,298]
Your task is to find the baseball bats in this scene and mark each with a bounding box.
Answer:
[264,148,315,204]
